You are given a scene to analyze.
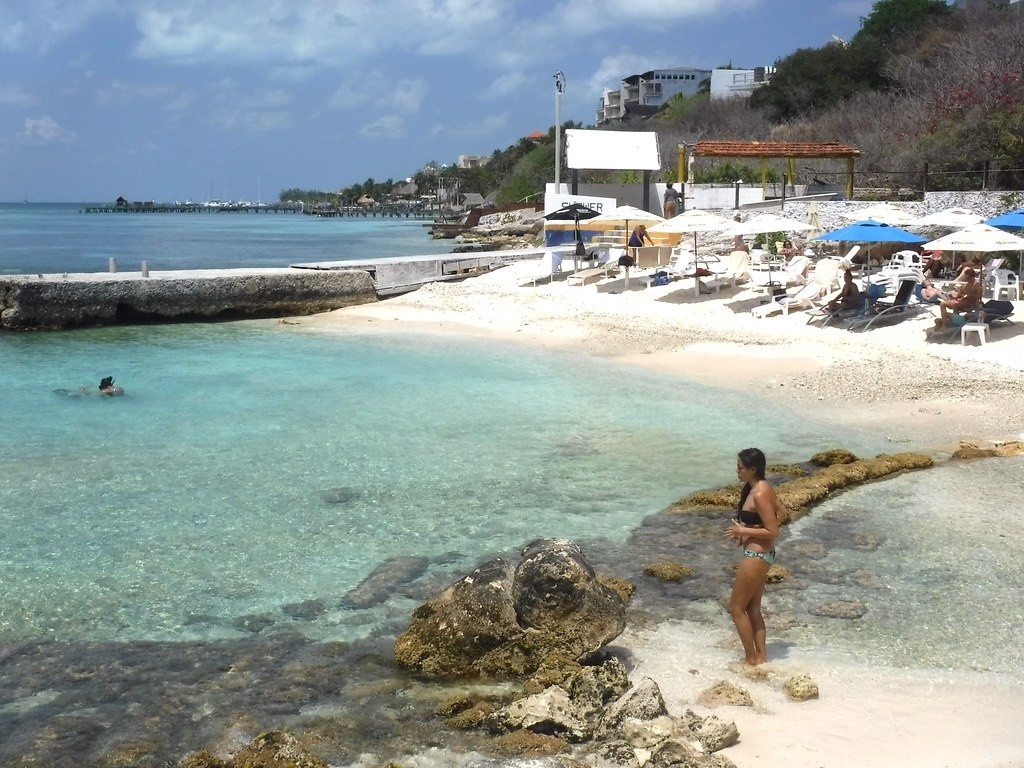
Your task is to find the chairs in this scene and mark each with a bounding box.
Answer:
[516,258,563,288]
[694,242,1021,338]
[566,263,614,288]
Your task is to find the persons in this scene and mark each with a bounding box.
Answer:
[936,269,981,331]
[724,448,786,667]
[629,225,653,262]
[663,180,679,219]
[921,250,944,277]
[735,235,744,251]
[753,238,763,250]
[953,258,985,281]
[779,240,797,263]
[766,238,777,261]
[827,272,859,317]
[922,278,964,301]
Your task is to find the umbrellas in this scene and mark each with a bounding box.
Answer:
[643,206,743,280]
[588,204,667,256]
[542,202,602,244]
[921,220,1024,302]
[809,216,928,299]
[719,212,814,286]
[839,200,919,264]
[908,205,985,270]
[982,209,1024,277]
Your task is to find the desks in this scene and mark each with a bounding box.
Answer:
[756,283,785,301]
[962,323,989,347]
[748,249,768,272]
[569,255,589,275]
[631,244,674,269]
